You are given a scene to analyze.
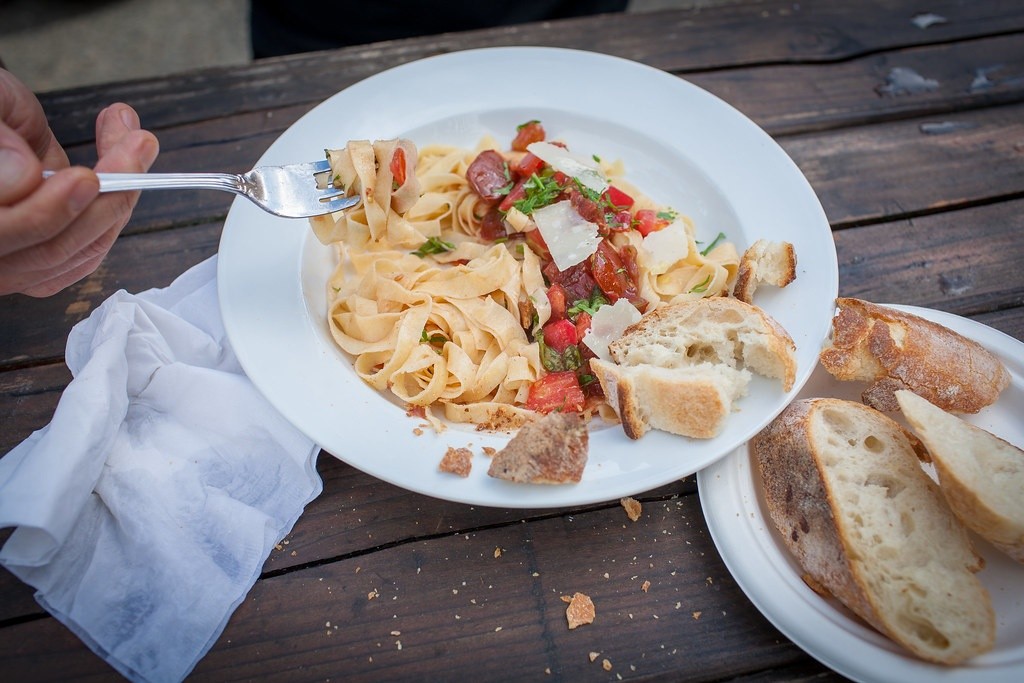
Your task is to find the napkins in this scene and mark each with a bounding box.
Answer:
[0,253,326,681]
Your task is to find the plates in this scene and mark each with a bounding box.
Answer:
[692,299,1024,683]
[213,41,840,515]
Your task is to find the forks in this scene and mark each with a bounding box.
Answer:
[35,155,364,222]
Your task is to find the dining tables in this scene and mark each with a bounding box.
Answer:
[0,6,1023,683]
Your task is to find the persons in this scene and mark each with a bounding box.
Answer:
[0,1,748,301]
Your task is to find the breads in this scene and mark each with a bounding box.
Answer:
[439,235,1023,664]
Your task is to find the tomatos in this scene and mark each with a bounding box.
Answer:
[391,122,668,414]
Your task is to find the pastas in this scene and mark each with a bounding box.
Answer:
[308,135,740,429]
